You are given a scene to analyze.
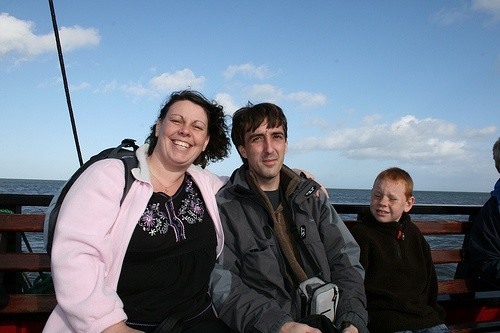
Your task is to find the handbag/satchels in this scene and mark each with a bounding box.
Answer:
[293,273,340,326]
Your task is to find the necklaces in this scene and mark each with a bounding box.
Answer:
[151,172,185,192]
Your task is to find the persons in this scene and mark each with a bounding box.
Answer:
[353,167,448,333]
[209,102,371,333]
[40,86,330,333]
[449,137,500,329]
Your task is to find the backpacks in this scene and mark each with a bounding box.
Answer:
[42,139,140,260]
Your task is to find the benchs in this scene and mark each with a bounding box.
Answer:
[0,212,500,333]
